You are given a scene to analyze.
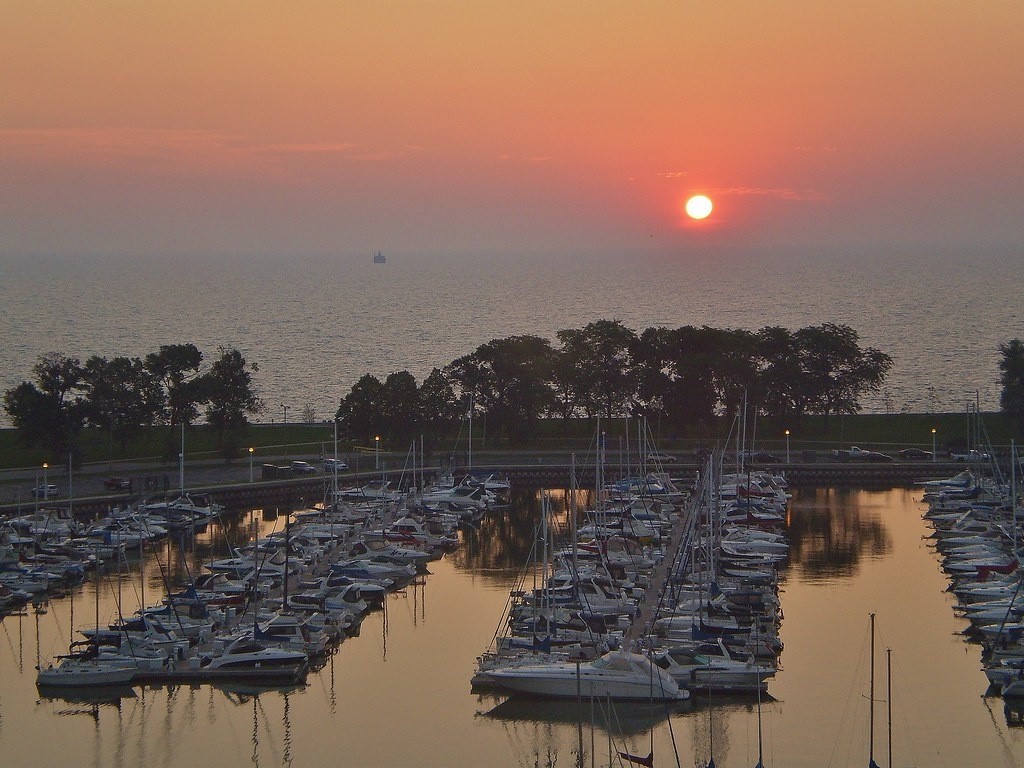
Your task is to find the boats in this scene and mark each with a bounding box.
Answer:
[0,492,224,615]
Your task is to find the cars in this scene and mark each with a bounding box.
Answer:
[32,476,172,498]
[262,456,350,478]
[645,445,993,465]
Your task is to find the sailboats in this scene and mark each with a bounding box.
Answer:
[470,389,794,705]
[914,389,1024,698]
[33,414,510,689]
